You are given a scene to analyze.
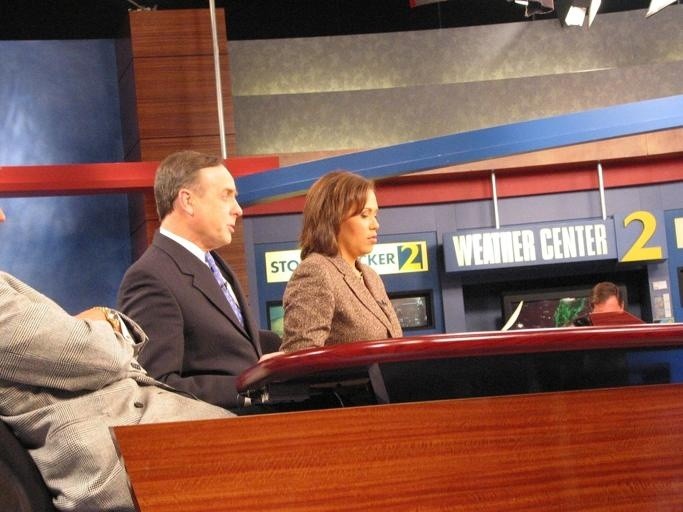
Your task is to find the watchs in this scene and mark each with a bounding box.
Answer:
[93,306,121,331]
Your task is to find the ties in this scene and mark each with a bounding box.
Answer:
[204,253,245,326]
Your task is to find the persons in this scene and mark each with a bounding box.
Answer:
[1,207,238,511]
[277,168,403,355]
[590,282,624,313]
[116,150,281,417]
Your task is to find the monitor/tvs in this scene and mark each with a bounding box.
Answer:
[502,285,629,328]
[384,289,436,330]
